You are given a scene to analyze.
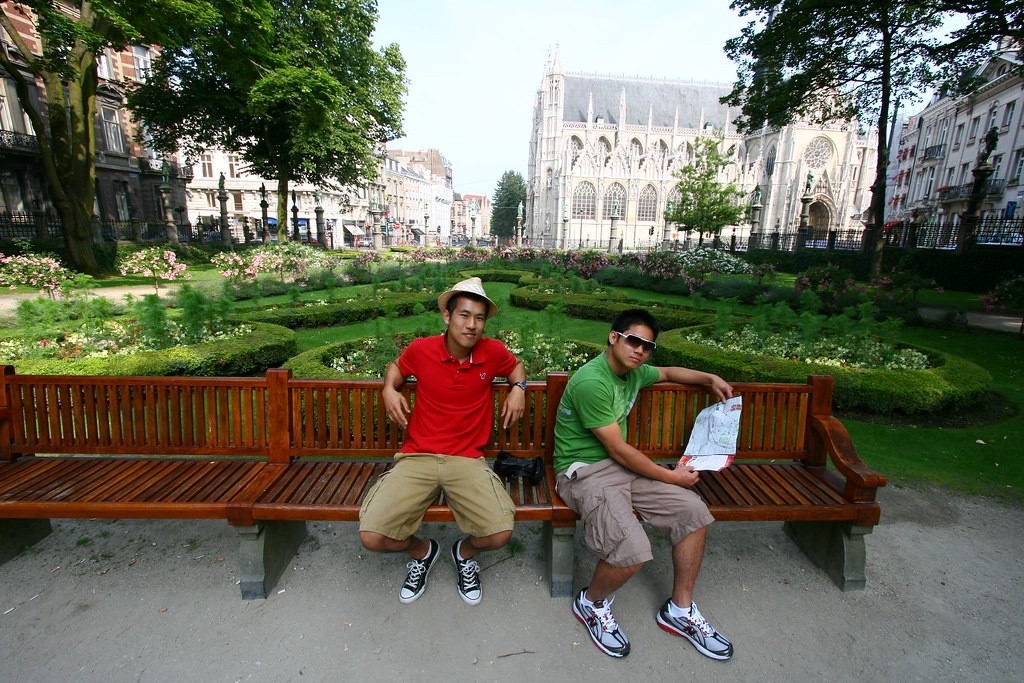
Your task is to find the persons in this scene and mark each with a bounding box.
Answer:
[358,277,526,607]
[552,307,734,660]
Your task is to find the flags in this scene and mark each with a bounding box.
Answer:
[384,209,390,217]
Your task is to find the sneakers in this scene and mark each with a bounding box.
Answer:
[450,539,482,605]
[656,598,733,660]
[572,587,630,658]
[398,539,441,604]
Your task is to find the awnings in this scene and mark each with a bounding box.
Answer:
[412,228,427,237]
[344,224,366,236]
[291,219,306,226]
[267,217,277,227]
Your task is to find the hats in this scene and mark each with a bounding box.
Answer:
[437,277,497,320]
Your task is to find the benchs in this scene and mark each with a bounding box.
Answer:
[0,365,888,593]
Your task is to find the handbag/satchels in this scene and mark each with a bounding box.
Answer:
[493,450,545,486]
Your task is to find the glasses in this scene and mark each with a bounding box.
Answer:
[615,332,657,352]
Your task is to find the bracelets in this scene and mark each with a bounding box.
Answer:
[512,381,527,391]
